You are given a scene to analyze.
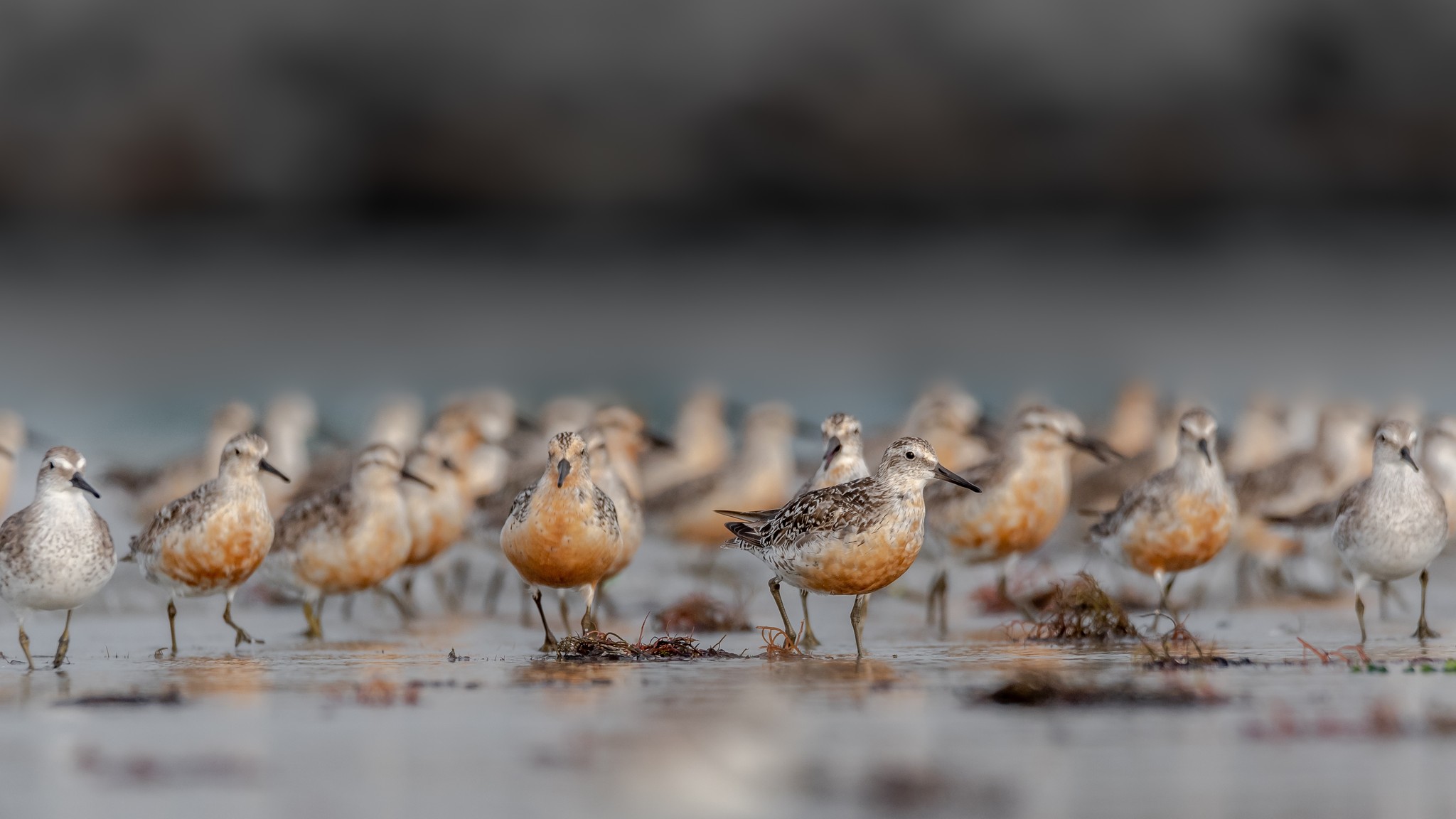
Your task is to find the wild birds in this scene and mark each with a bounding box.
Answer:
[0,379,1456,660]
